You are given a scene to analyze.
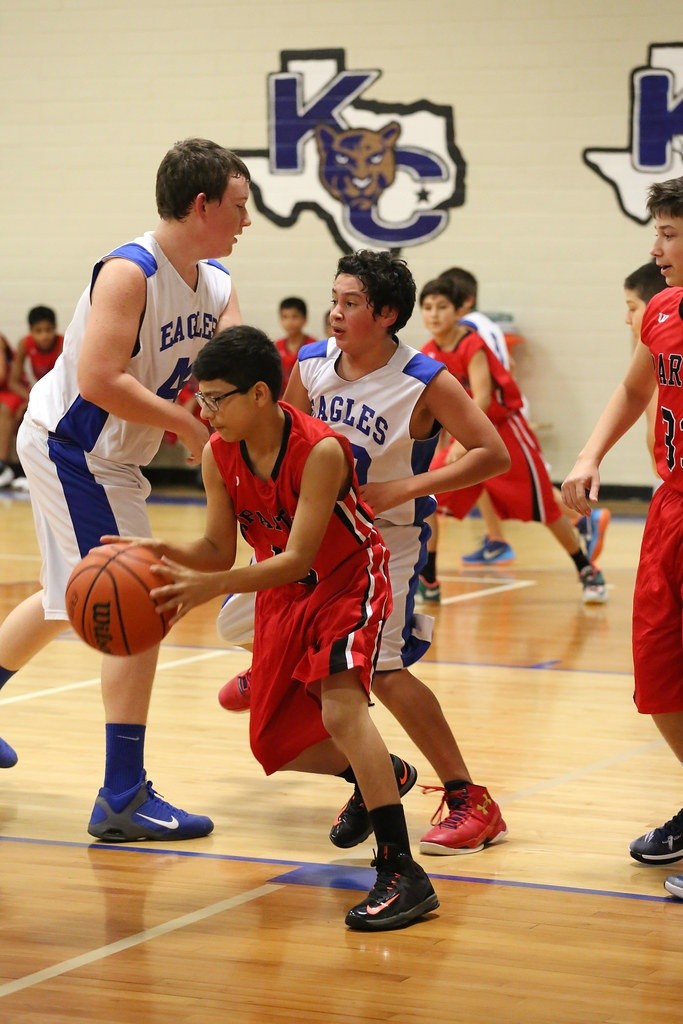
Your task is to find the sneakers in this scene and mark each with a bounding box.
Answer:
[217,667,253,711]
[0,464,14,488]
[630,808,682,865]
[461,535,515,566]
[87,781,214,843]
[662,875,683,899]
[345,857,440,929]
[419,785,509,856]
[585,508,610,562]
[582,571,606,603]
[414,575,440,604]
[329,755,417,849]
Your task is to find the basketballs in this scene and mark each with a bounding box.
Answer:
[65,543,180,657]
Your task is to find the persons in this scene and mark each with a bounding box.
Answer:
[414,268,613,605]
[100,324,442,934]
[216,250,507,855]
[561,176,683,900]
[0,305,69,494]
[0,138,252,842]
[271,296,320,394]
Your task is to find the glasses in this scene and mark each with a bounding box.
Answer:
[194,384,253,411]
